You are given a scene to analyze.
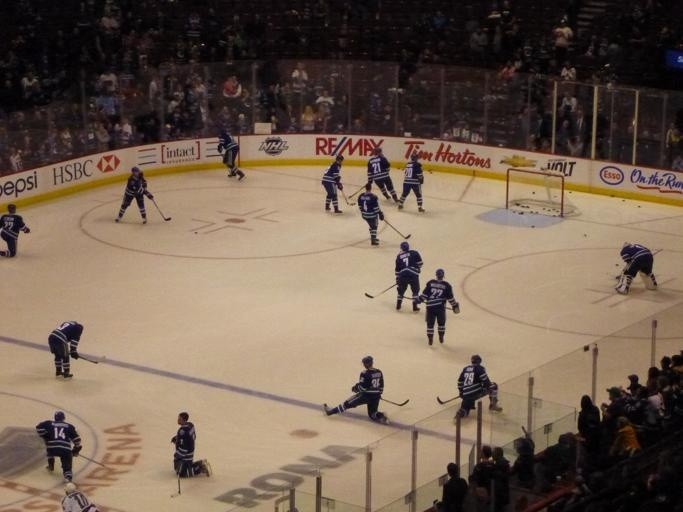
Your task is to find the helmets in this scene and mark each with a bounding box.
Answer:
[362,356,372,368]
[411,153,419,162]
[435,269,444,279]
[336,155,344,163]
[8,204,15,212]
[55,412,64,421]
[63,482,76,493]
[132,167,139,172]
[365,183,372,192]
[471,355,481,363]
[400,241,409,252]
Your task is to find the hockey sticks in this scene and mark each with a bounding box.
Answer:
[220,151,236,177]
[73,452,105,467]
[176,461,184,494]
[437,395,463,405]
[365,282,399,299]
[78,357,99,366]
[383,218,411,239]
[377,397,409,407]
[397,294,452,311]
[150,197,173,222]
[614,248,665,281]
[348,183,369,200]
[341,189,356,206]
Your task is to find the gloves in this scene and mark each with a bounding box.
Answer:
[144,190,153,199]
[72,352,78,359]
[72,445,82,456]
[378,211,384,221]
[336,182,343,191]
[23,227,30,233]
[415,295,423,304]
[452,303,459,313]
[218,143,223,153]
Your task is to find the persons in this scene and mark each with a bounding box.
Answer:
[48,320,84,379]
[615,240,659,295]
[0,1,682,180]
[114,166,154,226]
[170,412,212,480]
[394,240,424,311]
[320,153,344,213]
[434,351,683,512]
[398,153,427,213]
[358,184,384,246]
[412,268,462,349]
[61,481,99,512]
[0,202,31,257]
[366,148,401,203]
[322,356,386,422]
[35,411,82,481]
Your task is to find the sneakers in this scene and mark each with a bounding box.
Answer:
[55,371,73,378]
[0,250,10,256]
[323,404,332,415]
[326,203,342,213]
[115,214,121,222]
[385,193,403,209]
[397,301,420,310]
[45,465,54,470]
[455,410,465,418]
[199,459,209,477]
[418,207,426,212]
[372,237,380,246]
[376,412,387,421]
[142,218,147,224]
[228,167,245,181]
[428,335,444,345]
[490,404,503,411]
